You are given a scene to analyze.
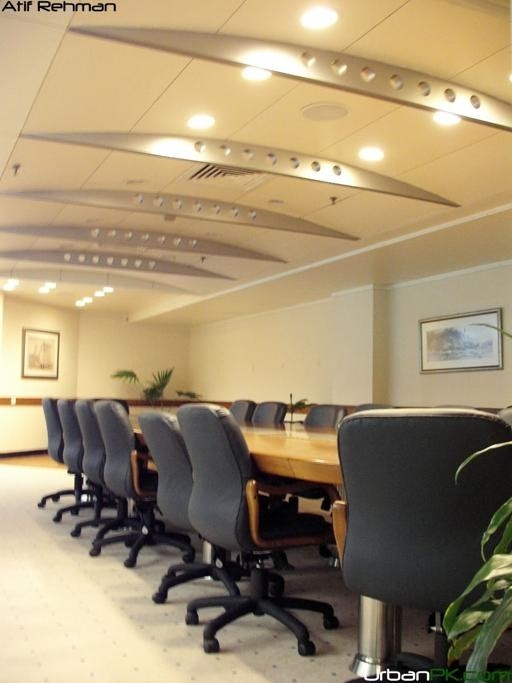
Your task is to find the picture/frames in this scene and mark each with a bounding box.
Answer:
[21,326,59,379]
[418,307,505,374]
